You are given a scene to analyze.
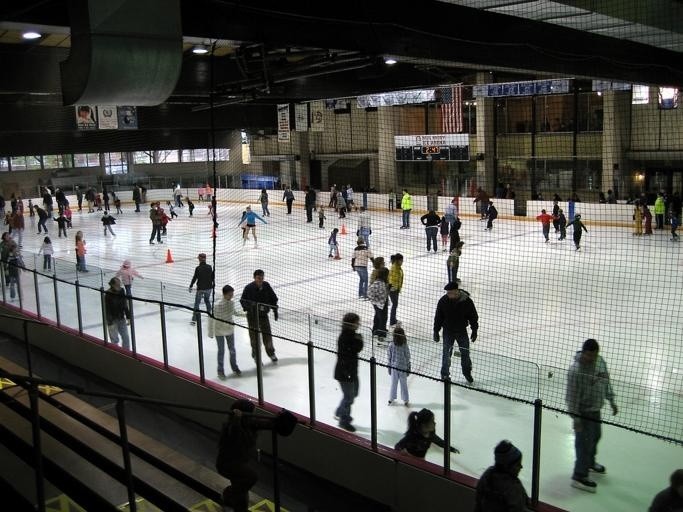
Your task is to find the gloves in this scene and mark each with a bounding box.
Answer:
[433,331,440,342]
[471,330,478,342]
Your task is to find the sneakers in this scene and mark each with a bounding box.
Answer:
[571,474,597,487]
[233,368,241,374]
[218,372,225,378]
[373,330,378,336]
[377,336,393,346]
[335,409,352,421]
[400,217,495,252]
[390,320,402,328]
[150,240,163,244]
[589,462,605,473]
[270,354,278,362]
[359,294,369,299]
[329,254,340,259]
[340,420,355,432]
[388,372,474,406]
[543,231,681,251]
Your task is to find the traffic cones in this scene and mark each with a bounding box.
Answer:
[332,247,341,261]
[339,222,346,235]
[208,226,217,240]
[164,249,173,264]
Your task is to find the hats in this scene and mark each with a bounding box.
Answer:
[403,188,407,193]
[444,282,458,290]
[495,439,521,464]
[123,259,131,267]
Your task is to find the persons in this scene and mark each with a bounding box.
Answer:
[447,242,464,283]
[497,176,683,252]
[76,105,95,129]
[333,311,364,433]
[74,184,147,236]
[392,408,460,466]
[238,184,358,260]
[1,186,72,302]
[388,183,496,253]
[75,231,87,273]
[476,439,531,511]
[105,260,145,350]
[563,337,620,492]
[216,398,259,512]
[366,184,377,193]
[351,206,404,346]
[648,469,682,511]
[149,182,220,244]
[386,326,412,407]
[188,252,279,379]
[434,282,479,384]
[120,110,134,126]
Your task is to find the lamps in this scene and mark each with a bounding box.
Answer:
[193,45,208,54]
[22,31,41,39]
[384,57,397,64]
